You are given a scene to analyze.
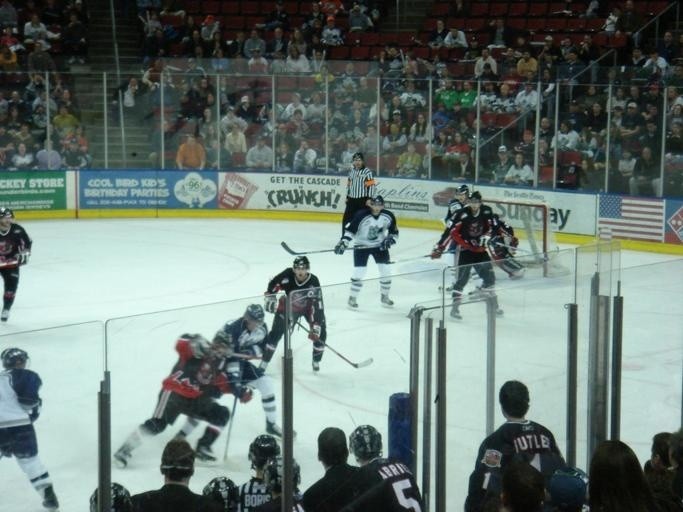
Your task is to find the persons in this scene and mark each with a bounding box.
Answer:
[113,330,252,469]
[1,1,98,172]
[177,304,297,441]
[0,348,60,511]
[90,425,425,512]
[0,208,32,321]
[335,195,399,309]
[111,1,683,200]
[261,256,326,372]
[451,191,504,319]
[463,381,683,512]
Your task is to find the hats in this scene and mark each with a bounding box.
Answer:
[548,473,584,509]
[160,441,194,470]
[319,429,345,454]
[352,152,362,161]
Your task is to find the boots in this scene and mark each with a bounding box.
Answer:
[197,440,218,462]
[115,449,131,463]
[42,487,59,507]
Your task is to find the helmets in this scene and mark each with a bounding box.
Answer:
[90,481,134,512]
[371,195,384,207]
[349,426,381,458]
[204,434,300,512]
[245,306,265,328]
[208,329,235,351]
[1,347,26,367]
[456,185,491,216]
[1,208,13,220]
[294,256,310,270]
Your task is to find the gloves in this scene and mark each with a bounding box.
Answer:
[430,244,445,258]
[380,238,395,249]
[265,302,279,313]
[17,251,30,264]
[310,324,321,340]
[333,241,346,254]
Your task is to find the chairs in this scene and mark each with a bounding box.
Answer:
[0,0,93,170]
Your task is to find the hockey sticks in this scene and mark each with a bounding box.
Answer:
[281,241,381,255]
[223,358,250,468]
[278,312,373,368]
[382,246,479,265]
[494,242,559,261]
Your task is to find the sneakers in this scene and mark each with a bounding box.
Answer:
[265,424,282,437]
[348,296,357,306]
[2,309,10,321]
[450,310,462,320]
[495,304,503,313]
[312,361,320,370]
[381,296,393,305]
